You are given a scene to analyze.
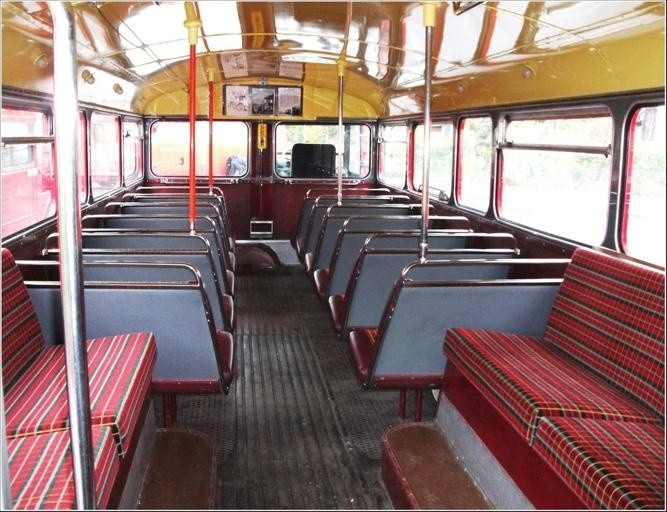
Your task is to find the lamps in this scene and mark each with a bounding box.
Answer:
[415,64,537,103]
[33,52,123,95]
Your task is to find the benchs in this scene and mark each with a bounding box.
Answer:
[2,246,157,510]
[13,186,238,425]
[435,246,665,510]
[290,187,572,422]
